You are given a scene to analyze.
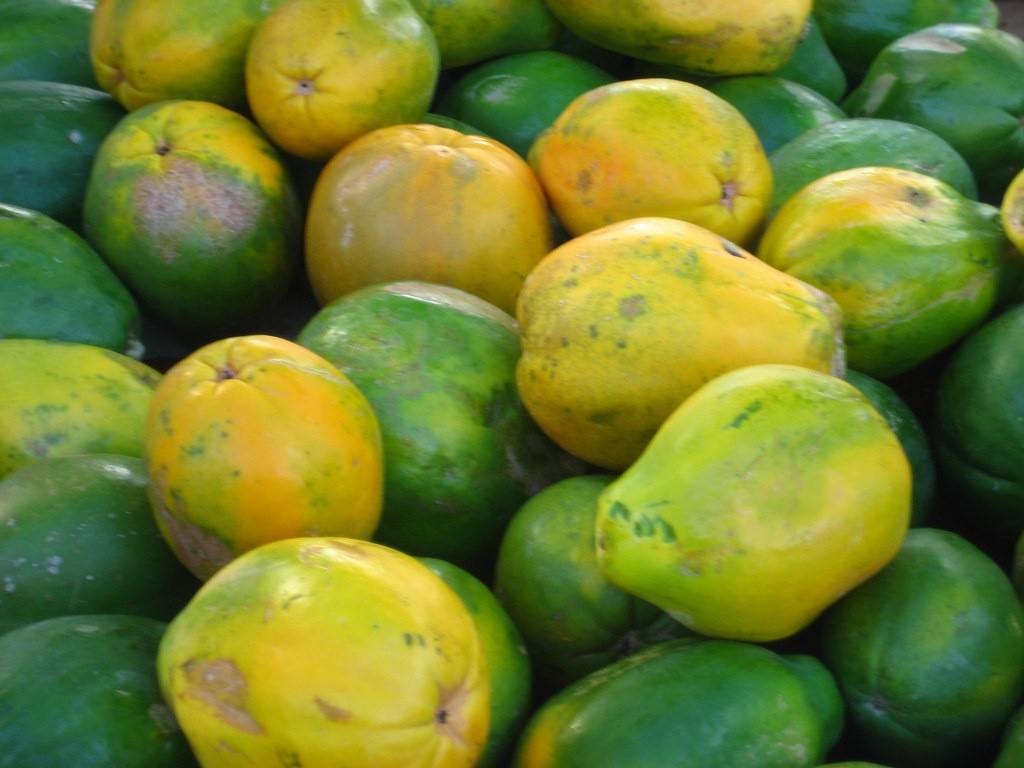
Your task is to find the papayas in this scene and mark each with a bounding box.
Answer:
[2,1,1024,768]
